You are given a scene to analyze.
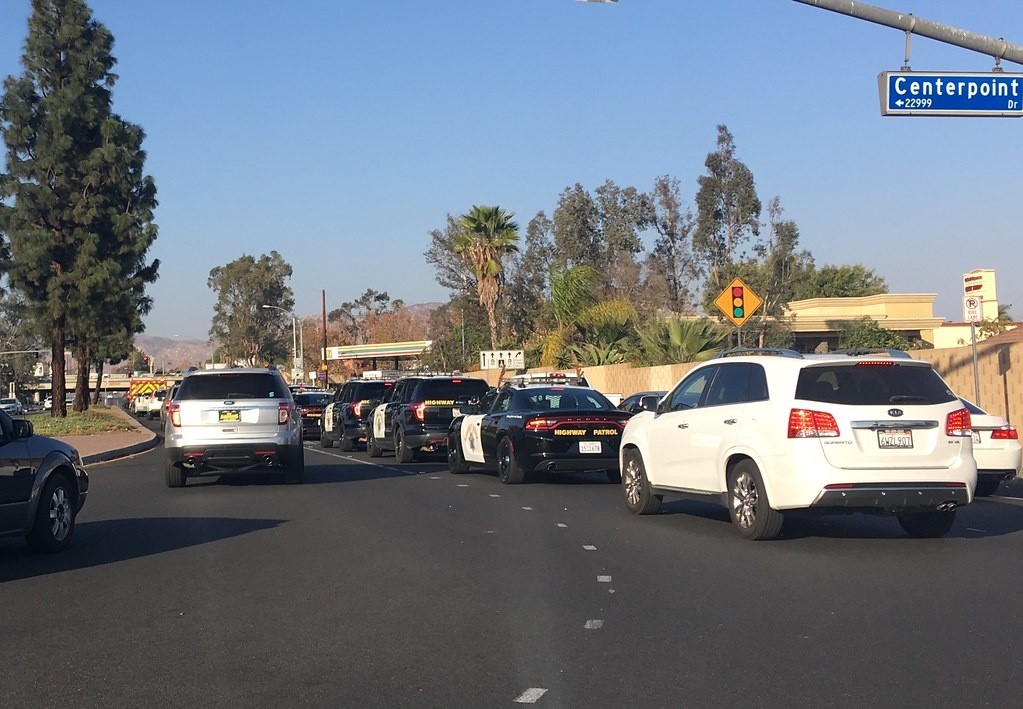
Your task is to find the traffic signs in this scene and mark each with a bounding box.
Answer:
[875,68,1023,117]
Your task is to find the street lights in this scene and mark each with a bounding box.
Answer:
[262,303,305,384]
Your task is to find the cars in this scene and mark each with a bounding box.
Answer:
[0,408,89,553]
[445,380,636,485]
[128,392,151,417]
[43,396,52,411]
[0,398,23,415]
[955,396,1023,498]
[616,390,720,415]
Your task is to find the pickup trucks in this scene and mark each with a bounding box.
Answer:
[503,371,625,408]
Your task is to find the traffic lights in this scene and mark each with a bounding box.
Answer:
[143,356,148,365]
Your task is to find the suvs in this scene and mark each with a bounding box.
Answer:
[318,378,395,452]
[363,375,490,464]
[164,362,310,487]
[291,392,336,440]
[145,389,169,420]
[618,345,979,537]
[158,385,180,432]
